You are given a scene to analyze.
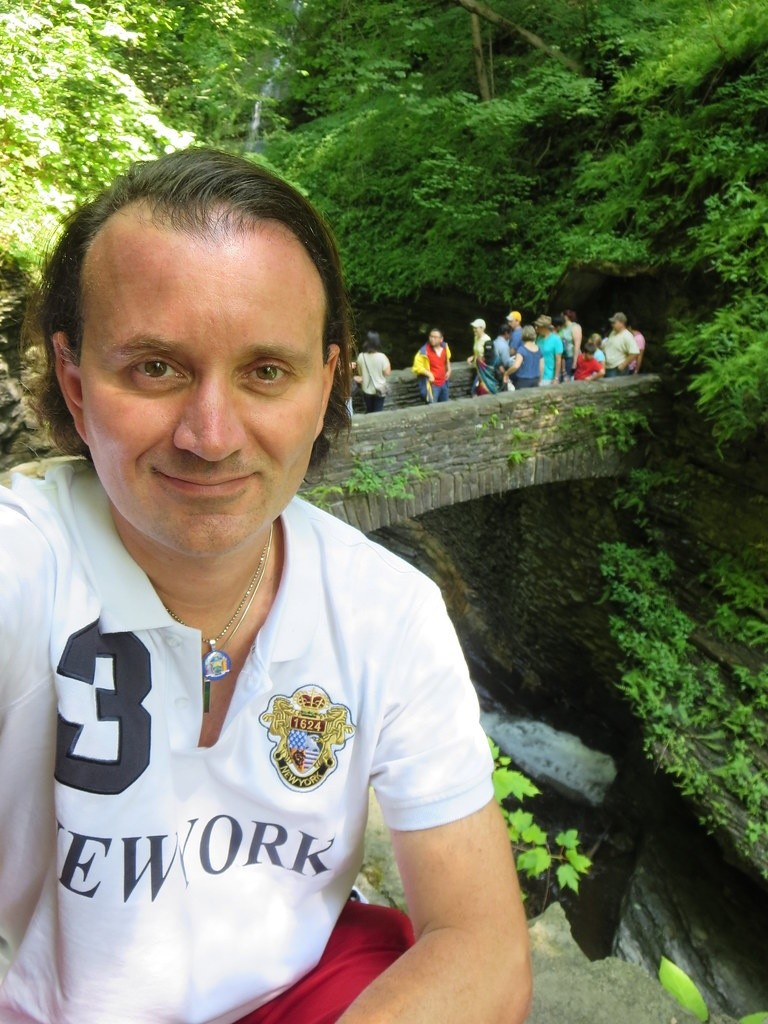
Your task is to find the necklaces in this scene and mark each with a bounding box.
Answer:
[166,525,272,714]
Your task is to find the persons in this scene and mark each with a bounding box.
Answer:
[467,309,645,398]
[357,331,391,414]
[343,362,363,418]
[412,328,452,405]
[0,145,533,1024]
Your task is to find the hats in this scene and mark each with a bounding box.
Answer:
[507,311,522,322]
[533,315,553,327]
[610,312,627,323]
[470,318,487,329]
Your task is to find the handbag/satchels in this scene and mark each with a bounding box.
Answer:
[502,380,518,391]
[372,378,393,398]
